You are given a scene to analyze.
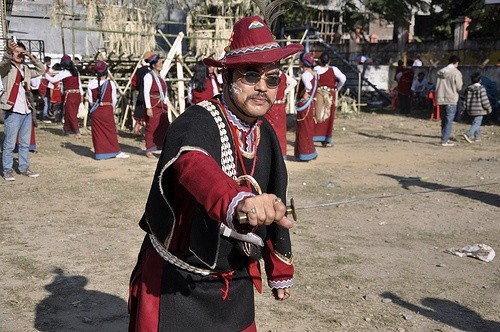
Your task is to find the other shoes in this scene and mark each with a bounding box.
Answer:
[474,137,481,142]
[323,142,332,147]
[441,140,454,147]
[73,134,81,139]
[146,152,156,158]
[462,133,472,143]
[116,152,130,159]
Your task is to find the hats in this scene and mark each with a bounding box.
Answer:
[61,55,72,64]
[145,54,158,63]
[302,52,314,66]
[94,61,106,73]
[203,15,304,68]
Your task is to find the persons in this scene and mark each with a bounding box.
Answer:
[390,53,435,118]
[313,52,346,147]
[88,62,129,159]
[129,15,303,332]
[0,38,45,181]
[460,72,492,143]
[131,52,223,159]
[295,52,318,163]
[495,59,500,66]
[42,54,83,139]
[40,56,96,123]
[262,69,297,161]
[435,55,463,147]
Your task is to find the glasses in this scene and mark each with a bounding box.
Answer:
[238,70,283,89]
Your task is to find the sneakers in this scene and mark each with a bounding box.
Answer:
[20,168,39,178]
[2,171,16,181]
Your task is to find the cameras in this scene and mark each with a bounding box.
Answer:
[11,35,16,45]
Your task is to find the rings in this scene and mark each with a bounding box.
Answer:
[246,208,256,216]
[272,198,282,205]
